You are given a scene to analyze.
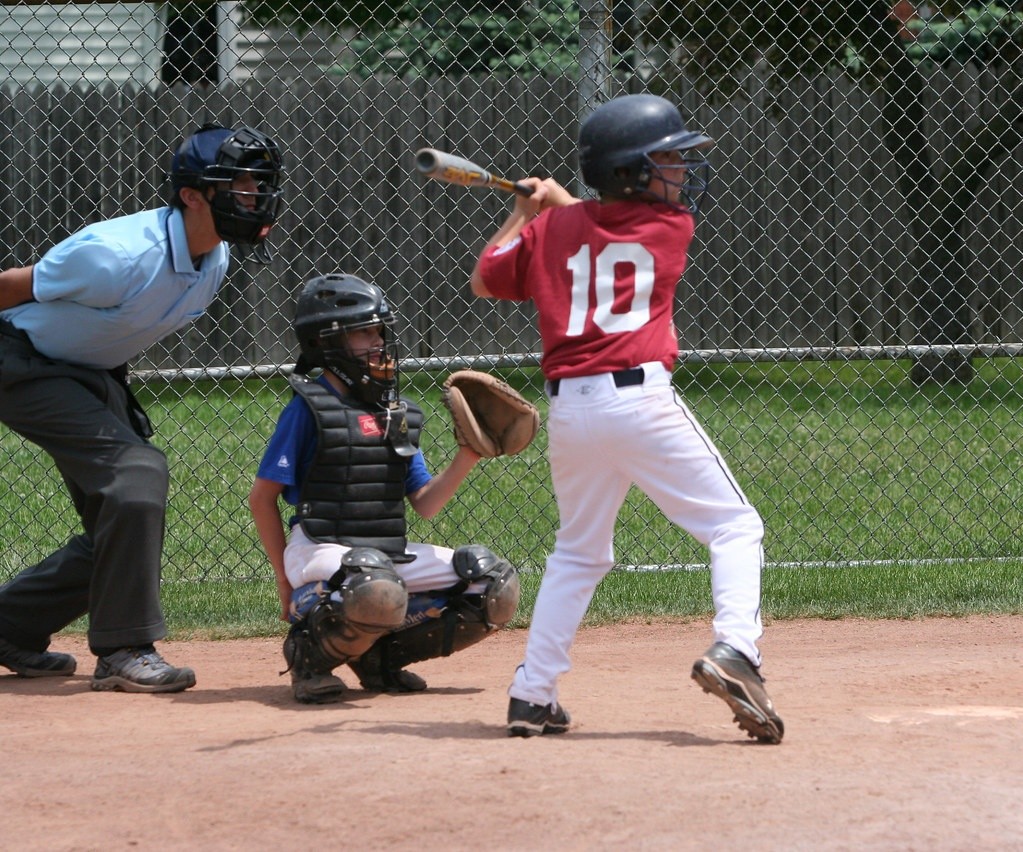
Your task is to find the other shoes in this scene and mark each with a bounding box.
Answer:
[282,643,337,703]
[347,658,414,692]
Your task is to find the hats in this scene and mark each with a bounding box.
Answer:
[171,121,264,188]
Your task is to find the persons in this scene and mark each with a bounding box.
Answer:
[470,95,784,744]
[247,272,520,703]
[0,122,286,690]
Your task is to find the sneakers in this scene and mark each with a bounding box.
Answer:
[691,640,785,744]
[91,643,196,694]
[1,638,77,677]
[507,696,570,737]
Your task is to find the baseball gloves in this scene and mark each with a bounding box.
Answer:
[440,369,540,459]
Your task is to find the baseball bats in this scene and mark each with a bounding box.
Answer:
[414,148,535,199]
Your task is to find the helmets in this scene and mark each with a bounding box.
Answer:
[295,273,397,409]
[579,92,713,197]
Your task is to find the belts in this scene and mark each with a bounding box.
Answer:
[551,367,644,395]
